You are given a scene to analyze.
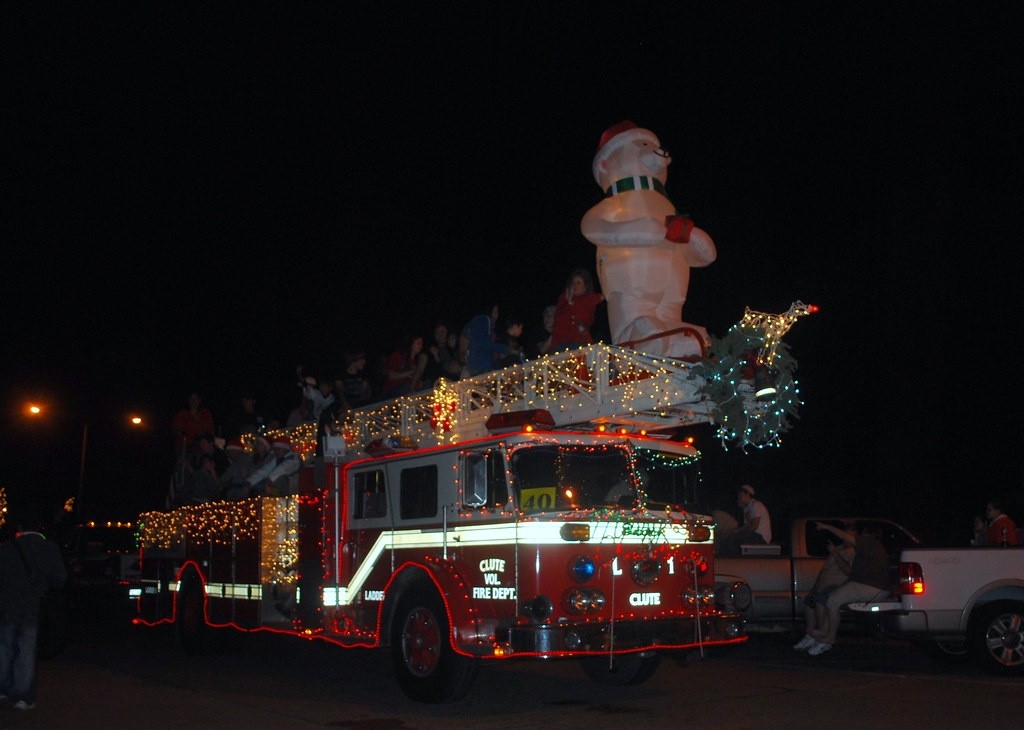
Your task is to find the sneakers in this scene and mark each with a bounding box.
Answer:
[808,643,832,655]
[794,635,815,649]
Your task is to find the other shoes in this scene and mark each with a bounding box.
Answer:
[0,695,9,702]
[14,700,35,711]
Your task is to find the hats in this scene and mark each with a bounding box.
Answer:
[226,440,245,453]
[741,485,755,495]
[259,436,294,451]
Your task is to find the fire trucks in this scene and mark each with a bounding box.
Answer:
[128,327,779,705]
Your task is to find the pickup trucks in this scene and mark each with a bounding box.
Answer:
[842,541,1024,674]
[706,514,932,654]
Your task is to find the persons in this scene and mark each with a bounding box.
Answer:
[0,513,67,711]
[378,304,527,402]
[285,342,372,428]
[713,484,772,558]
[974,497,1019,547]
[793,520,896,655]
[536,270,605,354]
[239,392,279,434]
[178,434,301,503]
[172,387,214,449]
